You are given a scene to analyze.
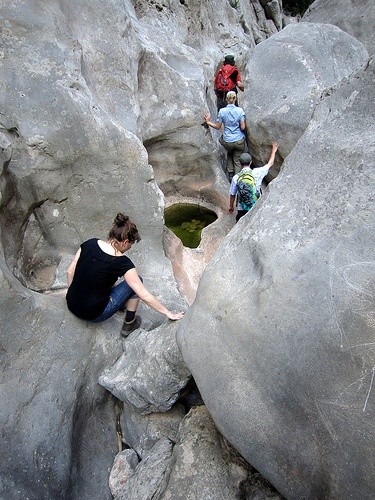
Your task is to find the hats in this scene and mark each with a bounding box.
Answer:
[223,55,235,66]
[227,91,236,99]
[239,153,251,164]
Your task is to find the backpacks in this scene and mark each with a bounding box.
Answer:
[236,169,256,206]
[216,68,238,94]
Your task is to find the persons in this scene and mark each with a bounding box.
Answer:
[204,91,246,182]
[228,143,279,223]
[214,55,244,111]
[66,213,185,337]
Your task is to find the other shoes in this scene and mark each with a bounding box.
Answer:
[119,305,127,312]
[120,314,142,337]
[226,167,234,173]
